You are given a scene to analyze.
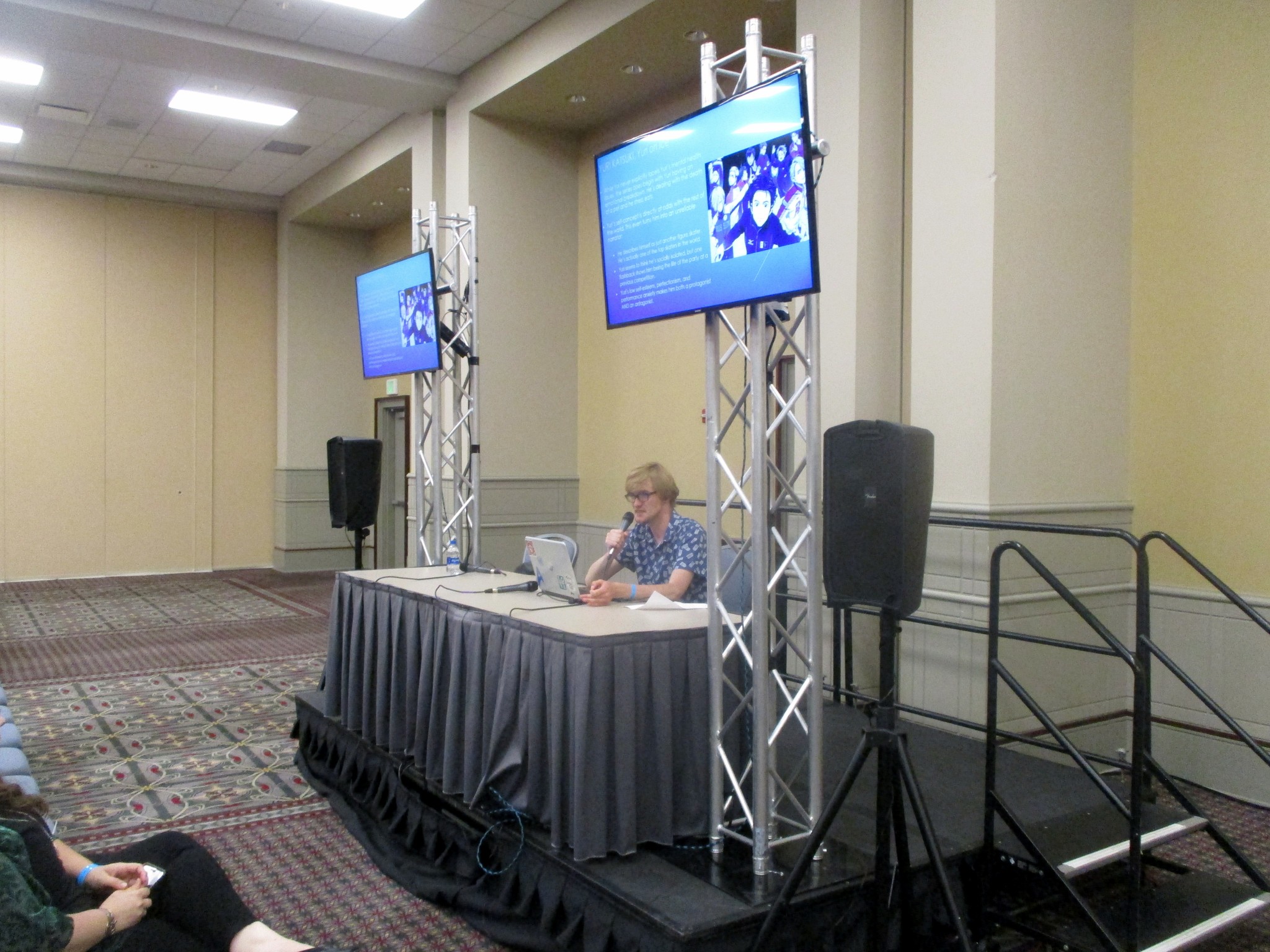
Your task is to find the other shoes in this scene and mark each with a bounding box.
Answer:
[298,946,362,952]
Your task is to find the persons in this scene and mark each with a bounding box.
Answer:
[579,462,707,606]
[0,778,328,952]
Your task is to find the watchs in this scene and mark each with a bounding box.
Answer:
[99,907,117,936]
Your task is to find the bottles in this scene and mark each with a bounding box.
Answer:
[446,541,460,575]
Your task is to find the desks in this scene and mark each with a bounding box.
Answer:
[325,566,749,866]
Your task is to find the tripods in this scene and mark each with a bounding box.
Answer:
[754,613,974,952]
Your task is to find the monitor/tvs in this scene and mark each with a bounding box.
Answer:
[356,248,442,379]
[593,65,821,329]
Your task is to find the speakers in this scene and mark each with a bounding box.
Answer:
[824,418,934,614]
[325,436,382,530]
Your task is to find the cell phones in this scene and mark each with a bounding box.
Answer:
[141,861,166,891]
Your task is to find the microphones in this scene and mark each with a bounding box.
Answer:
[459,563,502,574]
[608,512,635,559]
[484,581,538,592]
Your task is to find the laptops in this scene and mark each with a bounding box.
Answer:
[525,536,629,604]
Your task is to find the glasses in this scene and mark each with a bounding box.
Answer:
[624,491,656,503]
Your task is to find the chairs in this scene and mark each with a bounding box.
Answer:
[522,533,579,567]
[722,543,755,615]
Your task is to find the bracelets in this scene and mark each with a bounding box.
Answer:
[77,864,101,887]
[629,584,636,600]
